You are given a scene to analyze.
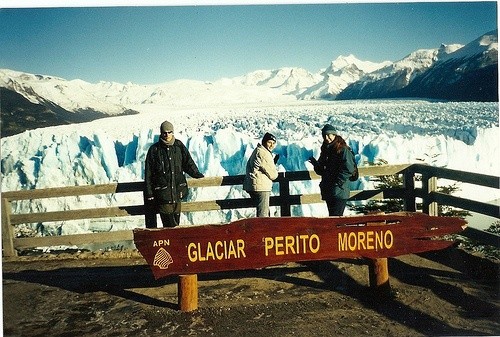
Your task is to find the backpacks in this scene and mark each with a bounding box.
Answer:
[327,145,358,182]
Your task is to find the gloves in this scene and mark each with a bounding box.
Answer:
[196,174,204,178]
[309,157,316,165]
[149,199,155,208]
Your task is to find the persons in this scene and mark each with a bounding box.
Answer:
[309,124,355,216]
[245,132,279,217]
[144,121,204,228]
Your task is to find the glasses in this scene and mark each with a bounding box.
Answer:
[162,131,171,135]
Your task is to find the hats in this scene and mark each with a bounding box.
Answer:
[262,132,276,145]
[322,124,337,135]
[161,121,173,131]
